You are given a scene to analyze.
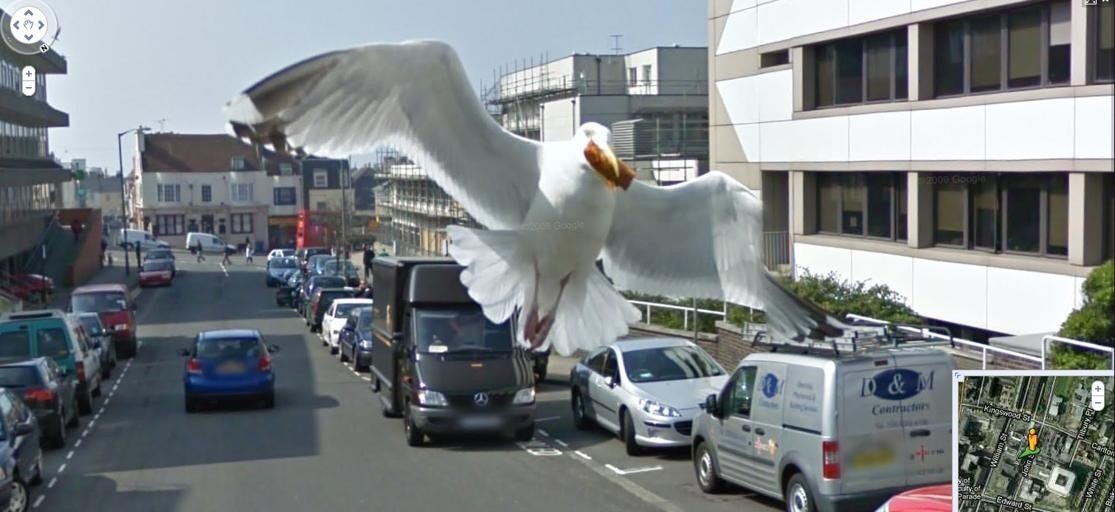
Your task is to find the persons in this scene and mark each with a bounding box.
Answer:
[363,243,375,277]
[246,242,254,264]
[379,247,389,257]
[135,241,141,267]
[72,218,83,241]
[196,239,206,264]
[102,237,107,260]
[222,242,232,265]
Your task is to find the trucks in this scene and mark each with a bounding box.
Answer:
[370,256,536,446]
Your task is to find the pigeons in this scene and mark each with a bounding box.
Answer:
[223,38,856,358]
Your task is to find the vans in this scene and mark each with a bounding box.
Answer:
[117,228,171,251]
[691,312,957,512]
[186,232,236,256]
[63,283,139,359]
[0,310,104,414]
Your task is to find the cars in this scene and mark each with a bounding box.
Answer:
[307,254,336,274]
[0,355,81,447]
[301,247,333,269]
[138,258,173,286]
[0,272,55,303]
[276,266,318,309]
[265,257,299,286]
[303,287,355,333]
[876,482,953,510]
[298,275,348,317]
[0,387,44,512]
[268,248,295,262]
[339,306,374,372]
[569,337,731,456]
[64,312,117,380]
[144,248,176,261]
[320,260,361,287]
[177,328,281,412]
[322,298,373,355]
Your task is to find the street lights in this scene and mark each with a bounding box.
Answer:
[118,126,151,277]
